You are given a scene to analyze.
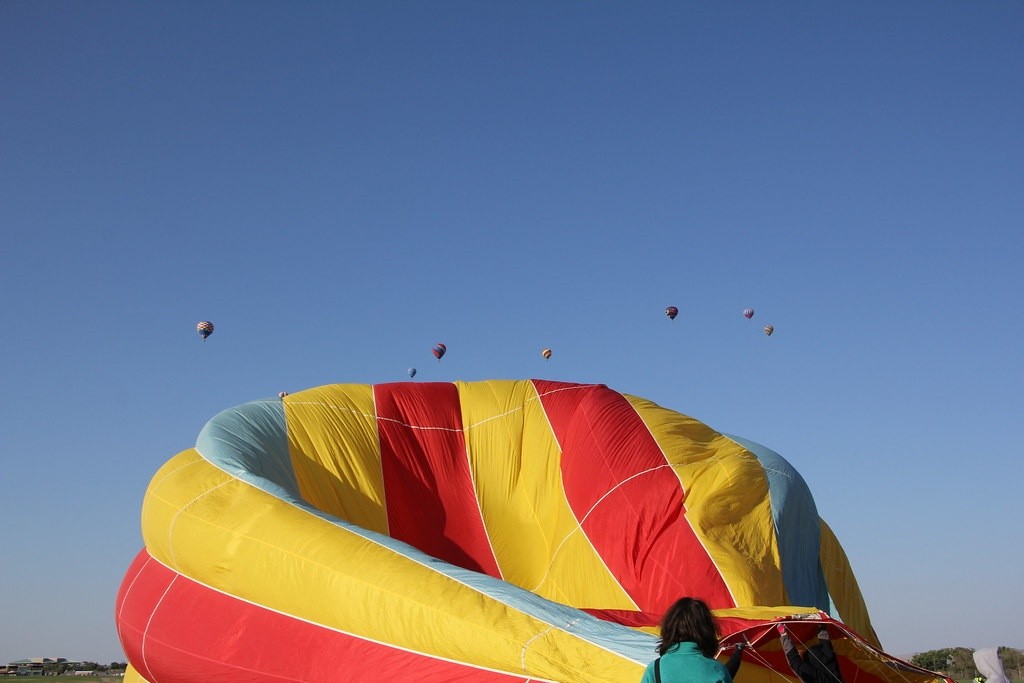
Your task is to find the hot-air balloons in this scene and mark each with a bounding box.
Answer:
[408,367,417,380]
[118,380,948,683]
[744,308,755,320]
[542,348,552,361]
[197,321,214,341]
[278,391,288,397]
[666,306,679,322]
[764,324,774,336]
[433,342,446,362]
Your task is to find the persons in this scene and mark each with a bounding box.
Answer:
[640,597,734,683]
[723,642,745,680]
[776,623,845,683]
[978,676,984,683]
[972,647,1011,683]
